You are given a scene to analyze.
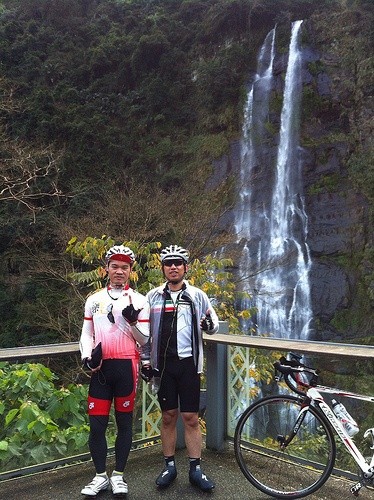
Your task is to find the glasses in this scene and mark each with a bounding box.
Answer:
[162,260,184,267]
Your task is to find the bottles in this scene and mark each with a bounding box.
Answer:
[332,399,359,437]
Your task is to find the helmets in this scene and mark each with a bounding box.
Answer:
[104,245,135,263]
[160,244,190,262]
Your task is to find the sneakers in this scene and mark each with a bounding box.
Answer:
[110,470,129,495]
[189,469,215,491]
[81,471,109,496]
[155,466,177,487]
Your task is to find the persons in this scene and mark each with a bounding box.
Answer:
[79,246,150,496]
[139,245,219,491]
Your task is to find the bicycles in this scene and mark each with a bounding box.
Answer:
[234,353,374,500]
